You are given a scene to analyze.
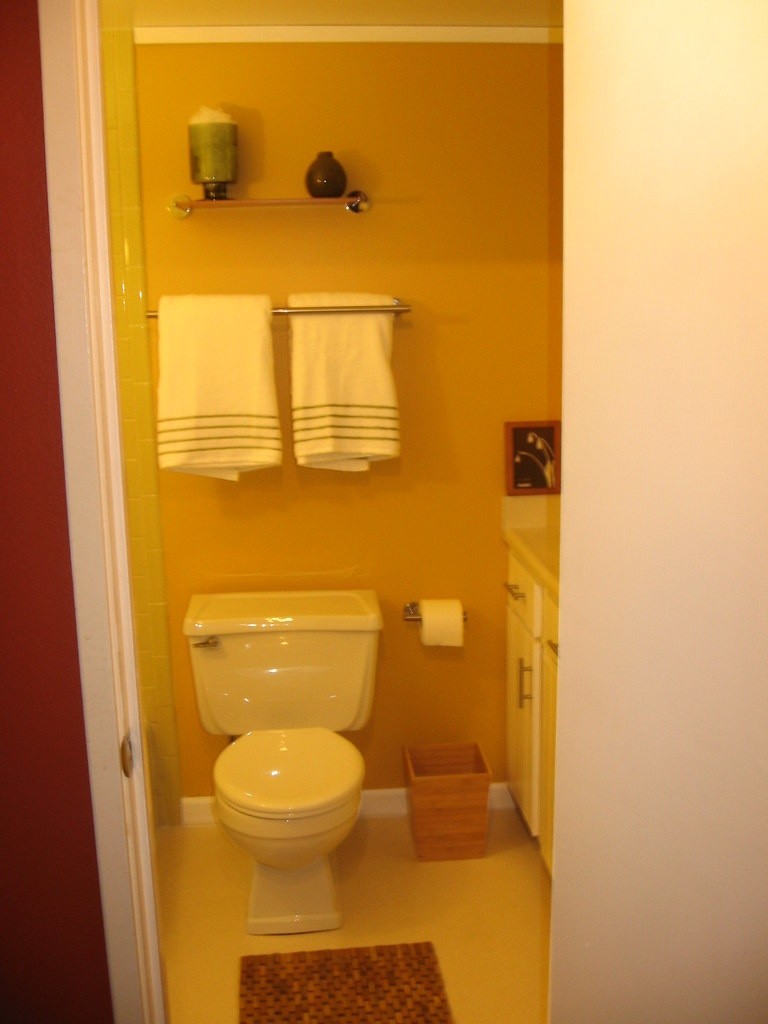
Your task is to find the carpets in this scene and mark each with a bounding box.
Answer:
[236,939,453,1024]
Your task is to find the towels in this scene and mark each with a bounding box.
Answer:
[287,292,401,473]
[155,293,283,483]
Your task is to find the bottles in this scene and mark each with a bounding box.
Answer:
[305,151,346,199]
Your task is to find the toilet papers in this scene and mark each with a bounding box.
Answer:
[416,597,463,647]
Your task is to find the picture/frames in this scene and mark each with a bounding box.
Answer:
[502,420,561,497]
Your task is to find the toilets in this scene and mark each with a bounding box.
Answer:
[182,590,385,935]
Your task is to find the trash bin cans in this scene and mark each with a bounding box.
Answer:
[401,742,496,861]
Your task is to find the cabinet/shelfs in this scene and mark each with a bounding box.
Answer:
[501,547,558,878]
